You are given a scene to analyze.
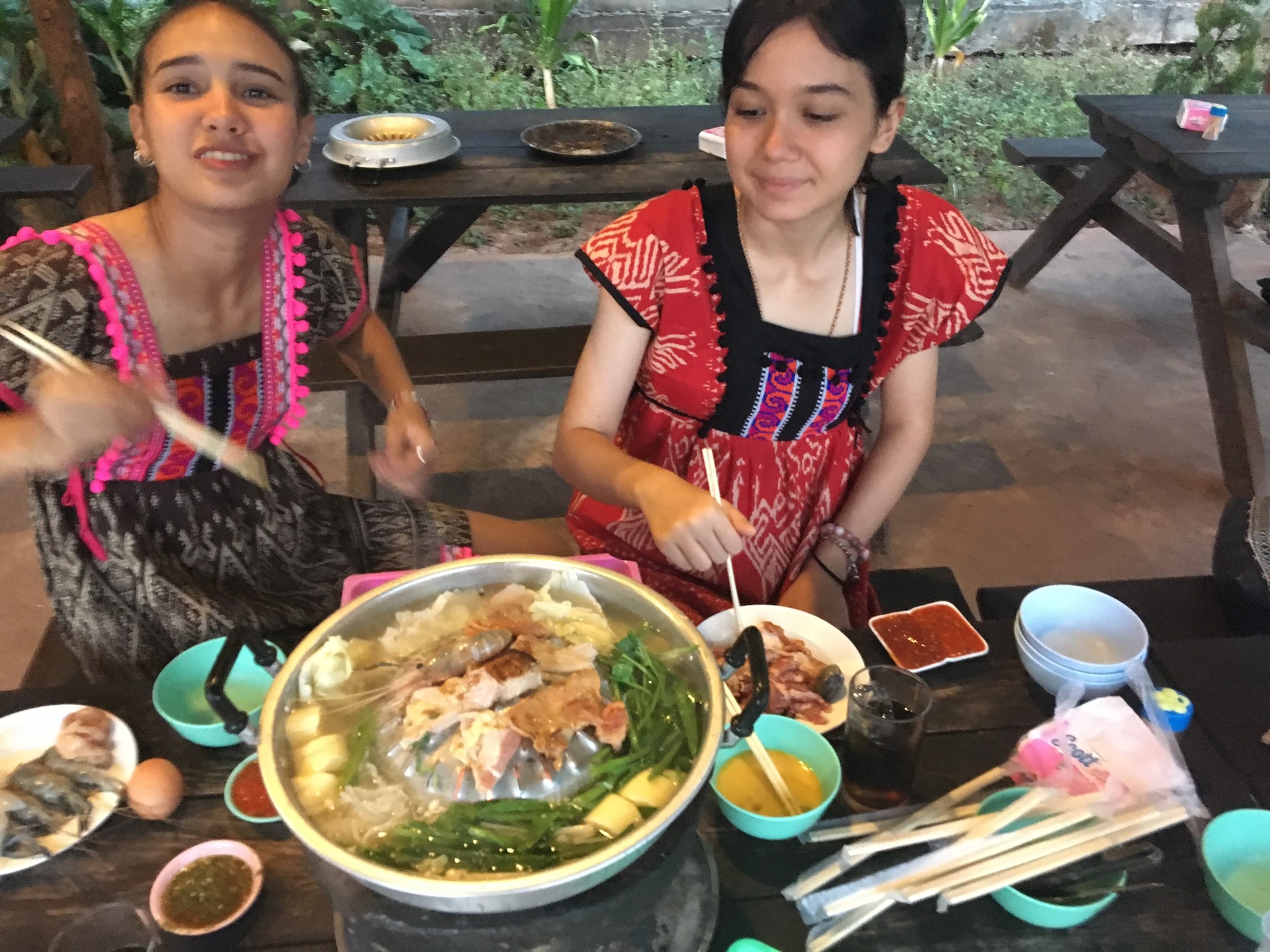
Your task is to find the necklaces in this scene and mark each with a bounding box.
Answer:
[830,212,854,343]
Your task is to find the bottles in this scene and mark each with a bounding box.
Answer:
[1201,105,1227,142]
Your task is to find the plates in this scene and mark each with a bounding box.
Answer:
[0,704,139,878]
[333,803,720,952]
[519,120,643,159]
[223,750,281,824]
[695,603,870,735]
[149,839,265,936]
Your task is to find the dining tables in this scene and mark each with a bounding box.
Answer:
[277,105,949,499]
[0,619,1270,952]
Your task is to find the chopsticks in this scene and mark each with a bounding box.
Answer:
[703,447,738,607]
[722,678,798,815]
[0,316,263,489]
[789,762,1191,949]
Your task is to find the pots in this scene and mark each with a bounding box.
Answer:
[204,553,770,915]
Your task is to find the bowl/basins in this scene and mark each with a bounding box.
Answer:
[1200,808,1270,944]
[322,112,462,188]
[869,601,990,674]
[1013,583,1150,699]
[706,713,844,839]
[151,635,287,748]
[973,786,1127,929]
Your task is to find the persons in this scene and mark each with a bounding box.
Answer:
[0,0,580,693]
[549,0,1011,645]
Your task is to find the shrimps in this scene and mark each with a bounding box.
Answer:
[320,625,514,717]
[1,747,129,856]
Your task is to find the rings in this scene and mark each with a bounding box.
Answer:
[418,446,426,463]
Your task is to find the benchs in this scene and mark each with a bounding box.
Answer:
[976,575,1270,665]
[0,165,94,244]
[296,320,984,500]
[18,568,982,691]
[1002,136,1105,293]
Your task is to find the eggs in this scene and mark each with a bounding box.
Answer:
[127,755,184,819]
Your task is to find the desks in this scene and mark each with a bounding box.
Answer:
[1006,95,1270,497]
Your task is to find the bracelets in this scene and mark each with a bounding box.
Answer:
[390,392,435,431]
[806,524,873,590]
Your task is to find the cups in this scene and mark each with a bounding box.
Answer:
[47,901,162,952]
[841,663,931,804]
